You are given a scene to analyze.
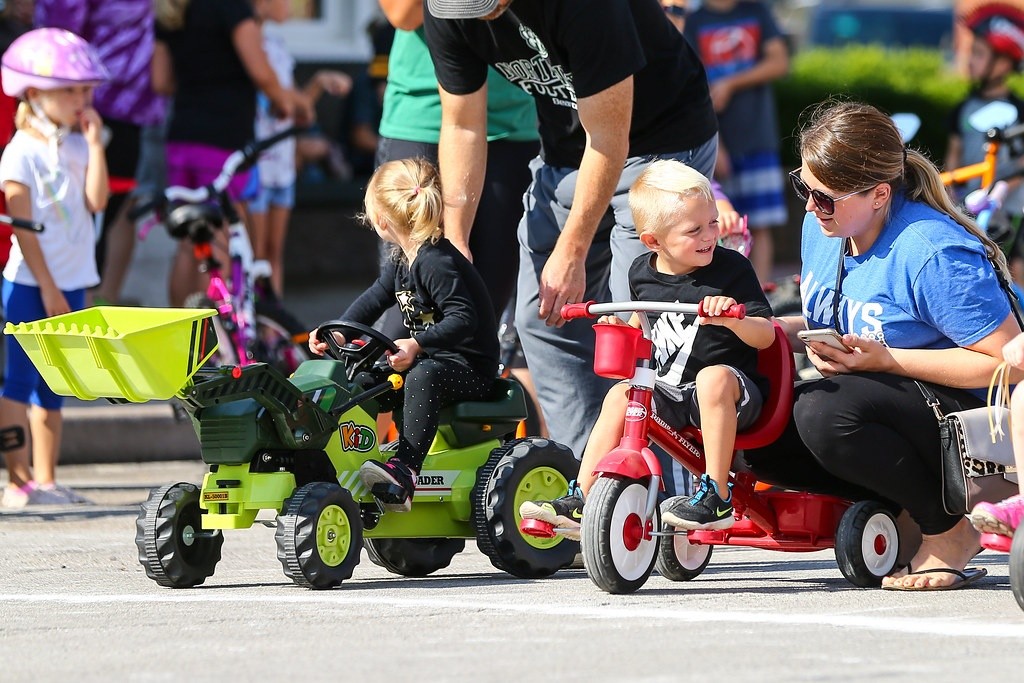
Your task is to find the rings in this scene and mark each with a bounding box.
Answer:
[566,302,571,304]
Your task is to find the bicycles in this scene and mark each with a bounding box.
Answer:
[123,120,324,379]
[935,123,1024,190]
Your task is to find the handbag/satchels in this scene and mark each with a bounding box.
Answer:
[941,404,1020,515]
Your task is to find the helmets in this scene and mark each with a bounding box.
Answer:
[1,27,112,98]
[955,0,1024,61]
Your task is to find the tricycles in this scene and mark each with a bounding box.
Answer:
[560,299,900,594]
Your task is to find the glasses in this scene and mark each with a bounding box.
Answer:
[789,167,879,215]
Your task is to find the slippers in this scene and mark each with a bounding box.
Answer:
[881,561,988,591]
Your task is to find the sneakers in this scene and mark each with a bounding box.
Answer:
[2,480,69,508]
[39,484,92,506]
[519,480,585,541]
[359,456,417,513]
[661,474,735,530]
[971,494,1024,539]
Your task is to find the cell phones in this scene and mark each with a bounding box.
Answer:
[797,329,855,362]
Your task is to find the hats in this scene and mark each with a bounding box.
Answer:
[427,0,499,19]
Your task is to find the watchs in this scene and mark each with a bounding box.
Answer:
[662,6,687,16]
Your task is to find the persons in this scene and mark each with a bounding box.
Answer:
[518,160,777,542]
[0,1,551,516]
[655,0,793,295]
[968,332,1024,540]
[421,0,722,571]
[942,0,1024,294]
[751,102,1024,593]
[307,156,501,514]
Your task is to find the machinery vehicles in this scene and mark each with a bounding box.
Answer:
[2,302,581,591]
